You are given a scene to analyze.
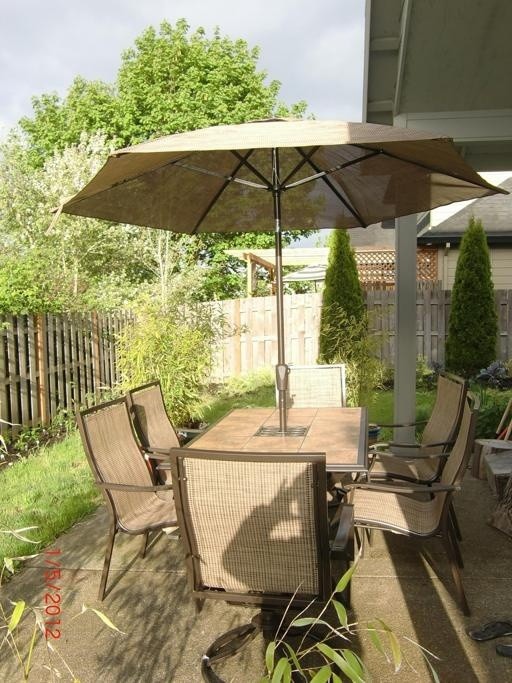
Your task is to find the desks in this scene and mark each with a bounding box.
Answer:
[154,405,370,558]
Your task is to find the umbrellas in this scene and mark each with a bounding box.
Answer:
[59,115,508,433]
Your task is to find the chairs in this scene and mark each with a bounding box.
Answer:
[330,391,480,617]
[275,362,347,406]
[367,369,472,540]
[127,379,206,483]
[78,395,179,602]
[169,445,355,682]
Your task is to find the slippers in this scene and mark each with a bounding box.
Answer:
[497,645,512,656]
[469,620,511,641]
